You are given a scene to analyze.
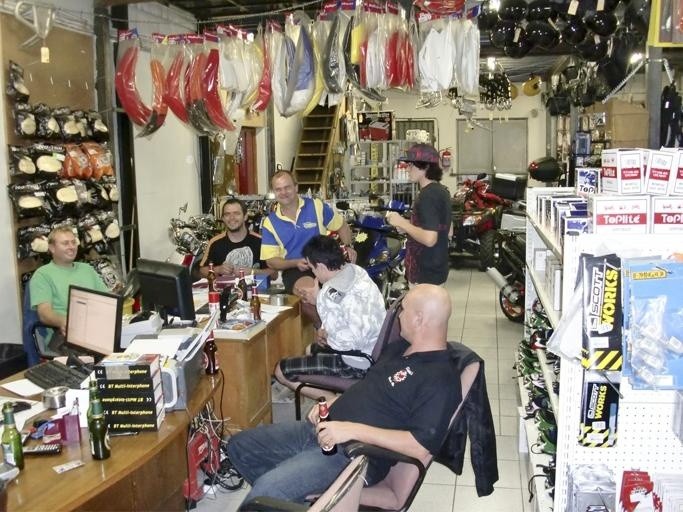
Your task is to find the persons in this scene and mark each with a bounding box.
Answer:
[385,143,454,290]
[260,169,358,329]
[198,198,279,281]
[273,234,387,403]
[227,283,463,512]
[28,225,111,353]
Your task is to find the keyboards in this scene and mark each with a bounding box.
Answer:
[24,359,86,390]
[195,293,238,313]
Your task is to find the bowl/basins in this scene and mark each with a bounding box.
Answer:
[122,298,135,317]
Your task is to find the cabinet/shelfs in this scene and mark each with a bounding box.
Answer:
[348,140,418,208]
[515,188,683,512]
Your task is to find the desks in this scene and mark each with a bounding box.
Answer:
[0,359,222,512]
[198,294,303,436]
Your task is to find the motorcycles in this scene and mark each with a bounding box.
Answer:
[170,172,414,311]
[445,171,526,323]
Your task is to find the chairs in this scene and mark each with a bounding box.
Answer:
[295,292,403,421]
[306,362,480,512]
[242,455,369,512]
[21,281,61,367]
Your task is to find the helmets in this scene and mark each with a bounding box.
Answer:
[396,145,439,163]
[387,200,405,214]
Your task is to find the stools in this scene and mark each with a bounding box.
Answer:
[183,423,221,501]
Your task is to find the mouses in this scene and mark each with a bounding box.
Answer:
[2,401,32,414]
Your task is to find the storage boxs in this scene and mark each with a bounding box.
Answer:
[585,97,649,149]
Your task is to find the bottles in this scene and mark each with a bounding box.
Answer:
[207,260,217,292]
[316,396,338,456]
[204,330,219,375]
[87,380,111,461]
[249,279,262,321]
[332,233,351,264]
[208,292,221,330]
[1,401,24,471]
[237,266,247,302]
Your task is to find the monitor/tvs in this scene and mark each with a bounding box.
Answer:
[65,283,124,365]
[135,258,196,322]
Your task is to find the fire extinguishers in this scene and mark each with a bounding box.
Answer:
[438,146,453,167]
